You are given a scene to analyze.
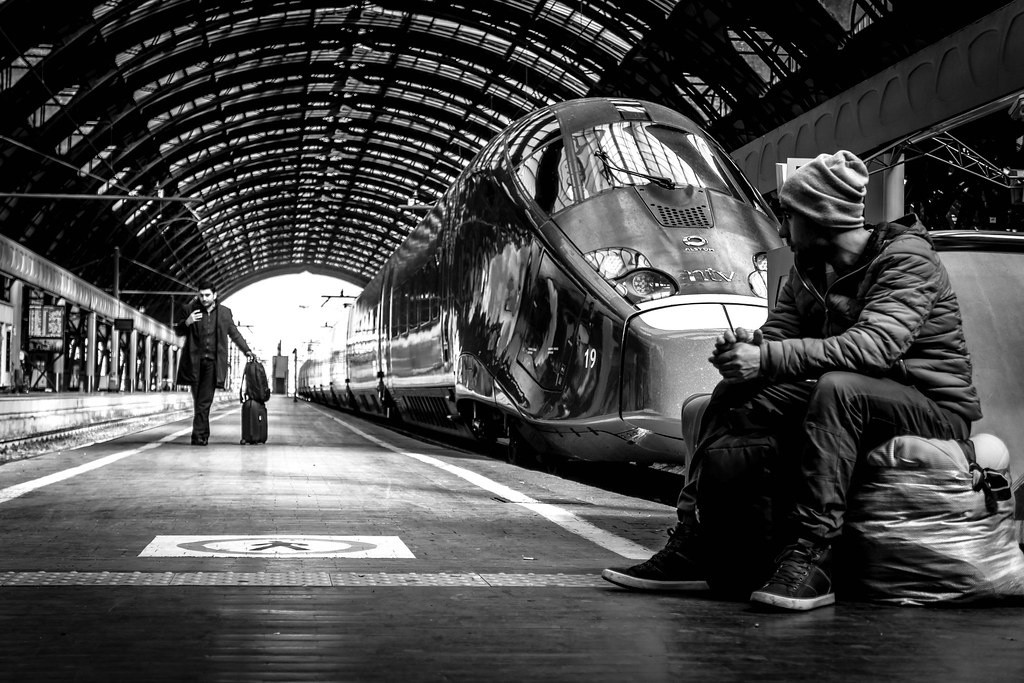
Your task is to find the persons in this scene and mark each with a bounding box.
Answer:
[173,281,257,445]
[602,150,984,609]
[531,278,598,421]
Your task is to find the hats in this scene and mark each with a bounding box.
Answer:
[780,151,870,229]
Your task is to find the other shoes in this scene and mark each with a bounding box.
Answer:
[191,436,209,445]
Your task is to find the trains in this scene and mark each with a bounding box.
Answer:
[297,96,797,466]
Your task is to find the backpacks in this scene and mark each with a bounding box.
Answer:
[240,356,270,403]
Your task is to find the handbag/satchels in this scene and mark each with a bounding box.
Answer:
[679,393,1024,606]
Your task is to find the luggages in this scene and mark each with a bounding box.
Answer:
[240,354,267,444]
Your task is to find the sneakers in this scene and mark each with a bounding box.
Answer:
[750,559,836,610]
[601,528,710,590]
